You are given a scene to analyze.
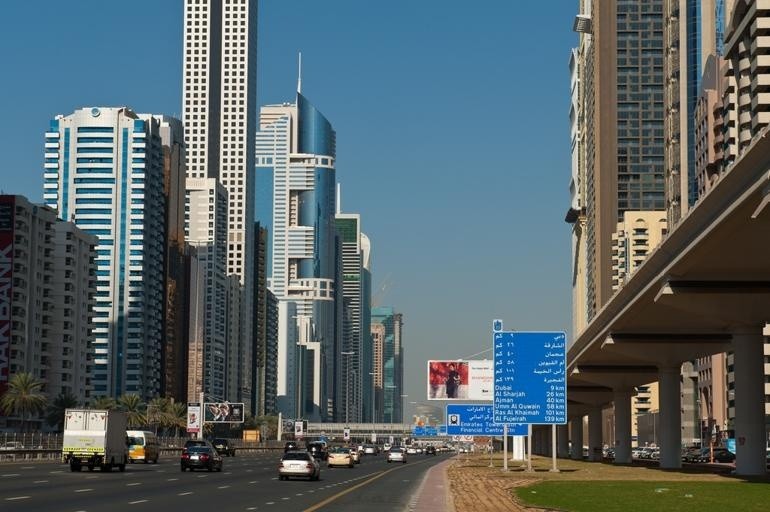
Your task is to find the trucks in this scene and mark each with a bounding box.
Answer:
[59,406,131,471]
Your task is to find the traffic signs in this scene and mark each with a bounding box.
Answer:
[447,403,529,438]
[493,332,568,427]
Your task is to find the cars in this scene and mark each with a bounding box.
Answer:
[1,442,24,456]
[380,442,474,455]
[386,447,409,464]
[579,441,770,468]
[362,443,377,456]
[325,448,356,467]
[276,451,322,481]
[348,447,361,464]
[178,445,223,472]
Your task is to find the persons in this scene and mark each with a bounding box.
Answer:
[208,404,223,421]
[445,364,460,398]
[218,404,230,421]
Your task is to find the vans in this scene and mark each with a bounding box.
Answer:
[124,430,161,465]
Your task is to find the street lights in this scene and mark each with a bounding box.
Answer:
[650,410,659,447]
[340,351,356,446]
[694,399,703,449]
[289,313,313,440]
[381,383,446,442]
[367,371,379,443]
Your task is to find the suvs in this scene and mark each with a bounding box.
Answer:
[181,440,212,458]
[284,441,329,466]
[212,438,237,457]
